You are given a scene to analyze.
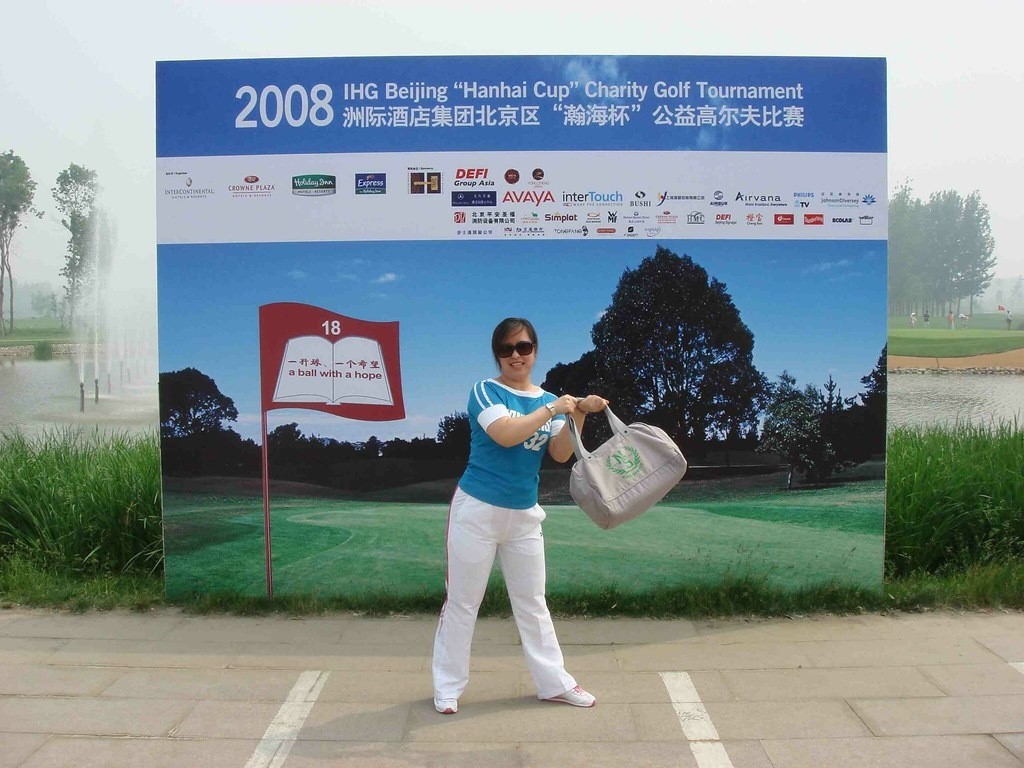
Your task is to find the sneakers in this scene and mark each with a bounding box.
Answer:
[543,685,596,707]
[434,698,458,713]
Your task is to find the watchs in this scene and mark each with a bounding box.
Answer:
[546,404,558,417]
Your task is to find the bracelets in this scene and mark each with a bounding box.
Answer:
[575,398,589,415]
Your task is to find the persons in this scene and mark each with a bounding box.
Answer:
[433,317,610,712]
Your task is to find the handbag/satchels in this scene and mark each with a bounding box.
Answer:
[566,397,687,529]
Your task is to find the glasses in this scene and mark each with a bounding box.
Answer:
[496,341,534,358]
